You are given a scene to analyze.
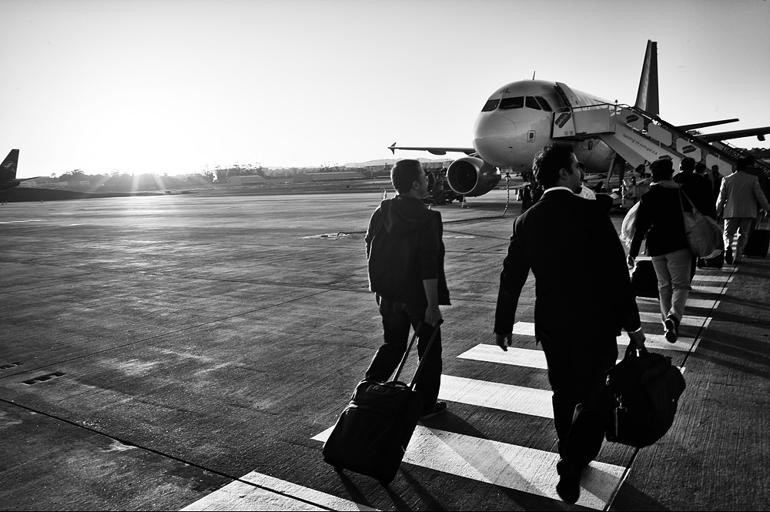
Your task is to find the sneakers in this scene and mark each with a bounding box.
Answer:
[662,317,679,343]
[725,246,733,265]
[556,458,581,503]
[734,260,742,265]
[421,399,447,419]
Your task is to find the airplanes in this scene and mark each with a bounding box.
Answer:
[387,39,770,238]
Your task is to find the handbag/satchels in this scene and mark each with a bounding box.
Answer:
[606,342,685,449]
[691,214,723,257]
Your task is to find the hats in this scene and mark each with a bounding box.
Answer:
[680,158,696,170]
[652,158,675,175]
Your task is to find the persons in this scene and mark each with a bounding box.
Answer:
[492,141,646,505]
[576,168,597,200]
[363,159,451,421]
[628,159,696,344]
[632,157,770,266]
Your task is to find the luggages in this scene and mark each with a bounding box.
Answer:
[698,240,724,268]
[744,212,770,257]
[631,260,660,300]
[322,319,444,490]
